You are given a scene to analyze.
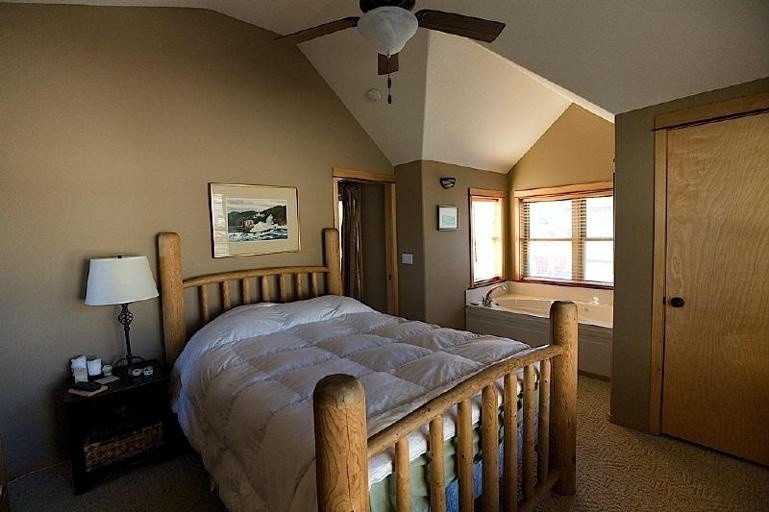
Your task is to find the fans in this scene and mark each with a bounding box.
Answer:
[272,0,506,77]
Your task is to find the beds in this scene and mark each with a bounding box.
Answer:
[155,227,579,512]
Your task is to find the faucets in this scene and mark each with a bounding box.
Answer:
[485,284,506,305]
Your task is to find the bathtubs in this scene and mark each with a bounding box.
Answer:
[466,294,613,330]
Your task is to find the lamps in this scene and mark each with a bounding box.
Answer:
[440,177,457,189]
[84,256,160,382]
[357,6,418,104]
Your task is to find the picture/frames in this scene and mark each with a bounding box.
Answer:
[208,181,302,260]
[437,204,461,231]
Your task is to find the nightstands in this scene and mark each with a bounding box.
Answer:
[63,358,179,497]
